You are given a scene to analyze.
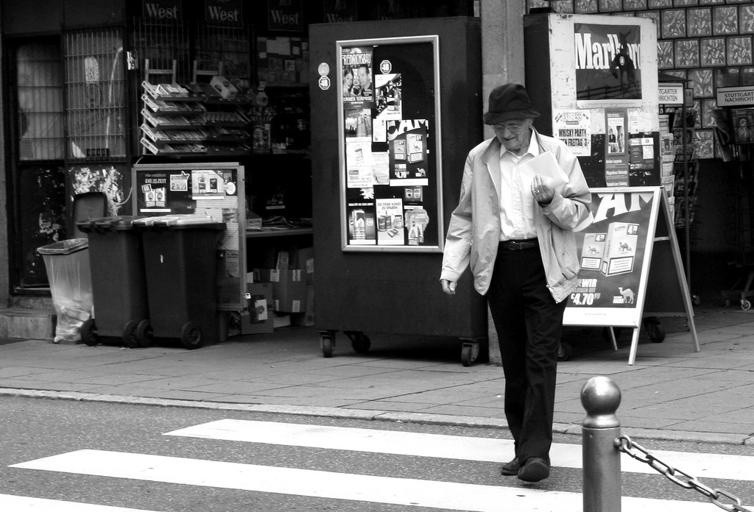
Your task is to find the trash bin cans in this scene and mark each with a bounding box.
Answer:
[39,192,107,345]
[132,224,226,349]
[75,215,148,345]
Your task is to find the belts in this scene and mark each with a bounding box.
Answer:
[500,240,535,252]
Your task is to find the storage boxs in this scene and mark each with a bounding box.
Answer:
[239,247,315,335]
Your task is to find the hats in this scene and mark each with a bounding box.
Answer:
[482,83,540,125]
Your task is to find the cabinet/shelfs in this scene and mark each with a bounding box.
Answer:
[137,79,254,159]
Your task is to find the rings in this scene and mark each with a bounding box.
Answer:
[535,190,539,194]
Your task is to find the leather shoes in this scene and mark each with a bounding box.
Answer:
[517,457,551,484]
[501,458,520,477]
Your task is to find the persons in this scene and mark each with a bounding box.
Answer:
[353,63,371,97]
[342,68,354,97]
[439,82,593,481]
